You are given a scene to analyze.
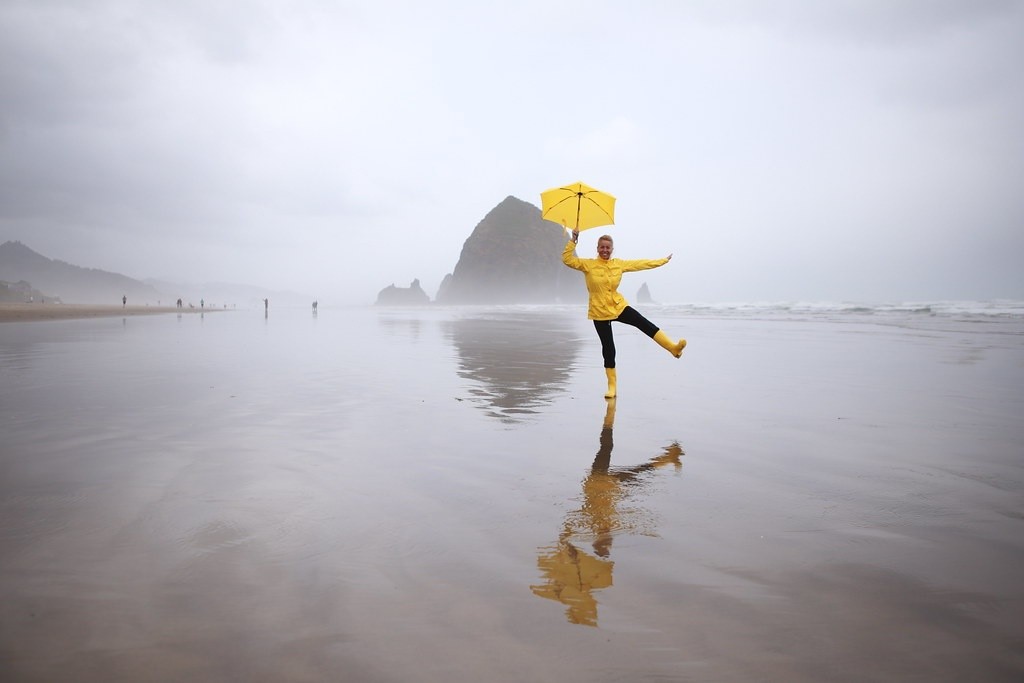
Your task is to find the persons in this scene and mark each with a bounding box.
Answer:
[264,298,268,308]
[201,299,204,308]
[562,226,686,398]
[312,301,318,311]
[122,296,126,306]
[177,299,182,307]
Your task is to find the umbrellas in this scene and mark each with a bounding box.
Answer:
[540,182,616,243]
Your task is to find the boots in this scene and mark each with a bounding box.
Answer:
[604,399,616,426]
[653,330,686,359]
[651,446,681,468]
[605,368,616,399]
